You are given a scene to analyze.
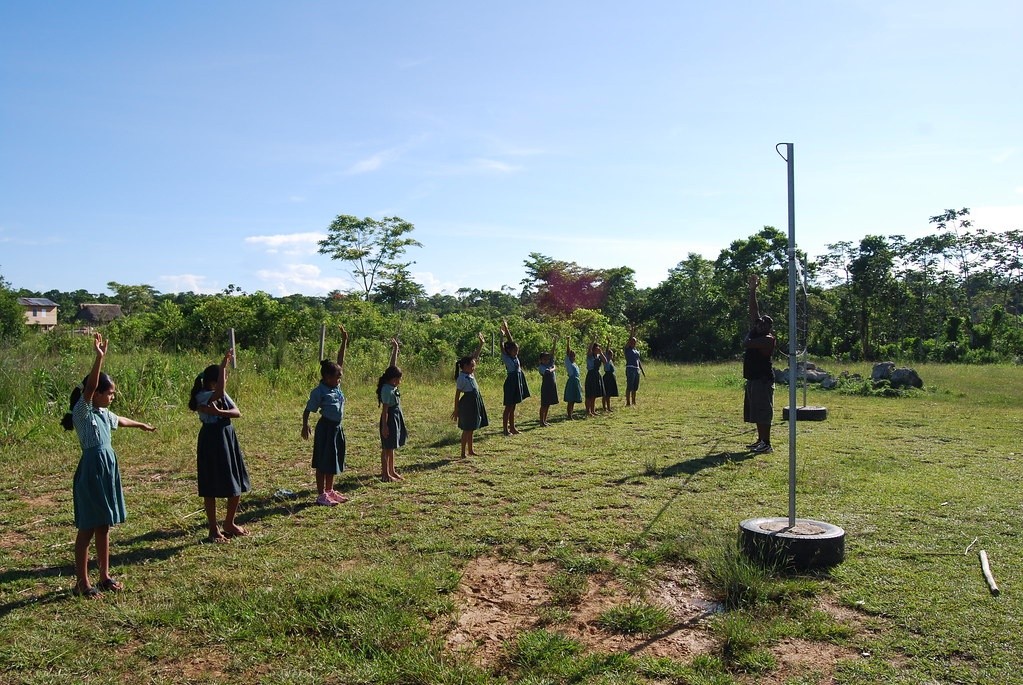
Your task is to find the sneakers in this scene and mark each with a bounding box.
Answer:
[317,488,349,506]
[746,439,774,453]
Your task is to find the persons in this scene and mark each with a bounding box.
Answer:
[625,326,645,406]
[743,274,776,453]
[449,333,489,458]
[563,335,582,420]
[584,335,606,417]
[60,332,158,601]
[188,347,249,544]
[499,320,531,437]
[375,338,409,483]
[300,323,349,506]
[596,336,619,413]
[538,335,559,427]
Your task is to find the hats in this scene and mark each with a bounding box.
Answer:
[758,315,773,325]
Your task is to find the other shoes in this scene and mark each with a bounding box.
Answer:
[627,400,639,406]
[540,421,551,427]
[587,410,599,417]
[223,526,250,536]
[603,408,614,413]
[210,530,231,544]
[503,428,521,436]
[566,415,578,421]
[75,578,105,600]
[461,451,478,459]
[382,473,404,483]
[97,578,125,591]
